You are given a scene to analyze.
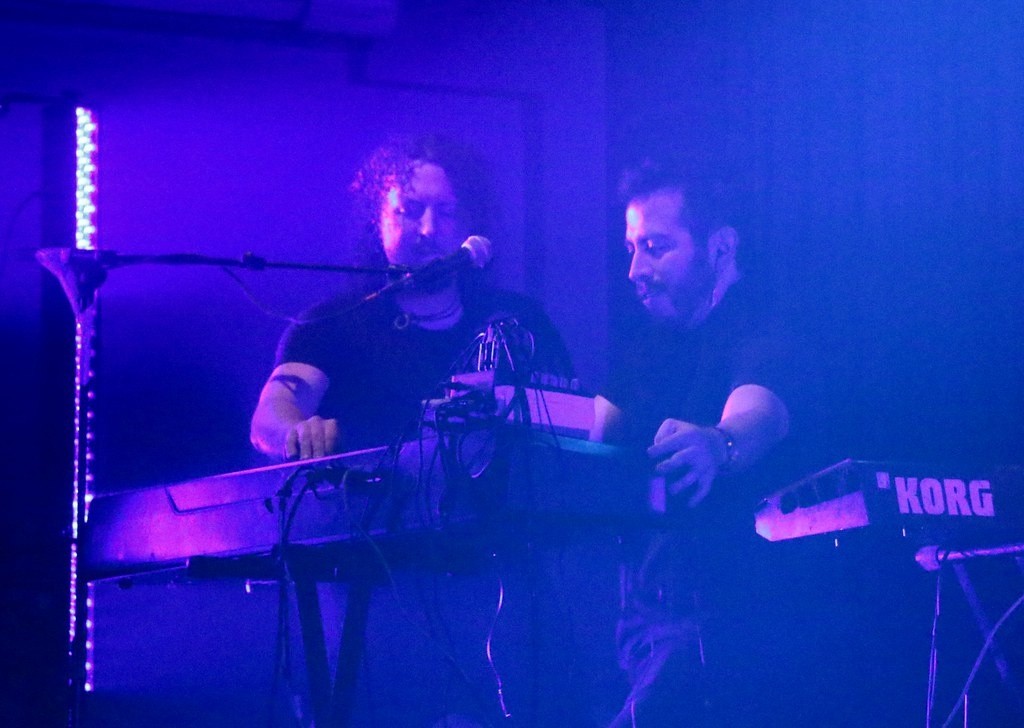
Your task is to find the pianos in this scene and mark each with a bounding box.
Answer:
[749,456,1024,728]
[75,418,739,728]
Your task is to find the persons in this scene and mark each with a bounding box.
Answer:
[249,132,583,728]
[585,161,860,727]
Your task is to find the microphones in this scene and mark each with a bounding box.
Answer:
[366,237,493,306]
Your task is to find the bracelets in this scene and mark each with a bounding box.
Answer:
[713,427,740,466]
